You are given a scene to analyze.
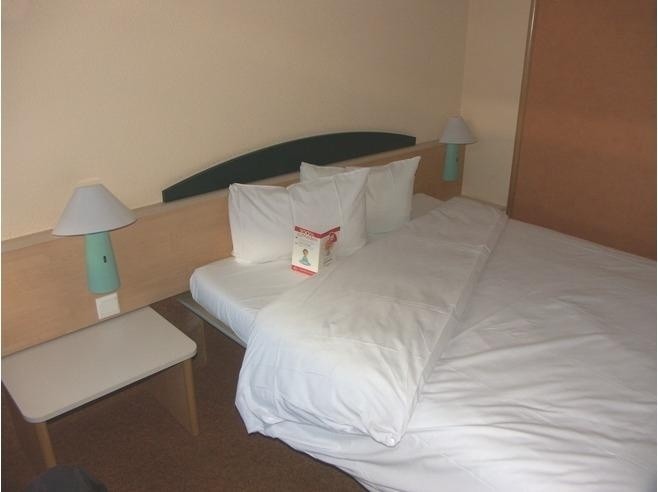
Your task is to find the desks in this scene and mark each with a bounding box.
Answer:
[2,307,199,470]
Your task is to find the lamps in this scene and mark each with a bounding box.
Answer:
[51,184,138,295]
[439,115,478,181]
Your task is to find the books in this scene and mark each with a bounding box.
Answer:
[290,225,341,277]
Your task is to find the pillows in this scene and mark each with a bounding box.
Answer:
[227,156,424,267]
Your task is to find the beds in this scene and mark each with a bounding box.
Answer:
[164,132,656,492]
